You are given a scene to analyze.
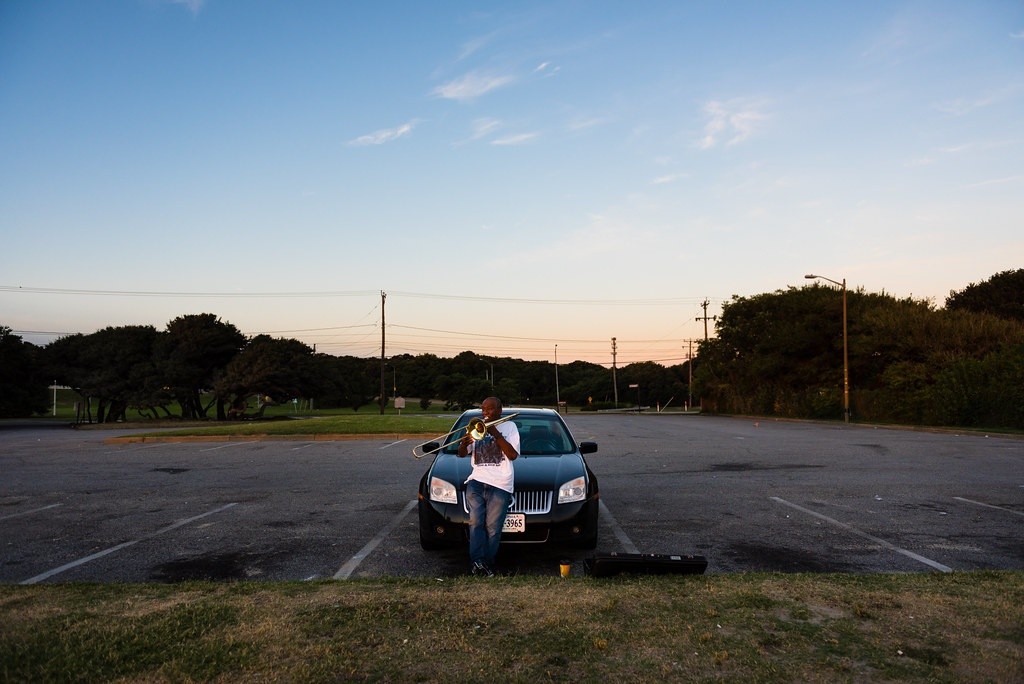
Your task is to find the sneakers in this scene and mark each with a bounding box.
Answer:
[467,561,495,578]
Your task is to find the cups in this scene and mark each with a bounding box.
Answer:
[559,560,571,578]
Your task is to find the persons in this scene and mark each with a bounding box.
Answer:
[457,396,521,583]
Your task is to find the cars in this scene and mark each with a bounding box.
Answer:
[417,407,603,552]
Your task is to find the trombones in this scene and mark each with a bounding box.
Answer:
[412,413,520,459]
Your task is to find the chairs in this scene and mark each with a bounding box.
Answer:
[522,426,555,450]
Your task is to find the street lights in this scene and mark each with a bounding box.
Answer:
[805,274,851,424]
[479,358,493,391]
[554,344,560,414]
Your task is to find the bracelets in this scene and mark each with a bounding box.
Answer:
[494,432,502,439]
